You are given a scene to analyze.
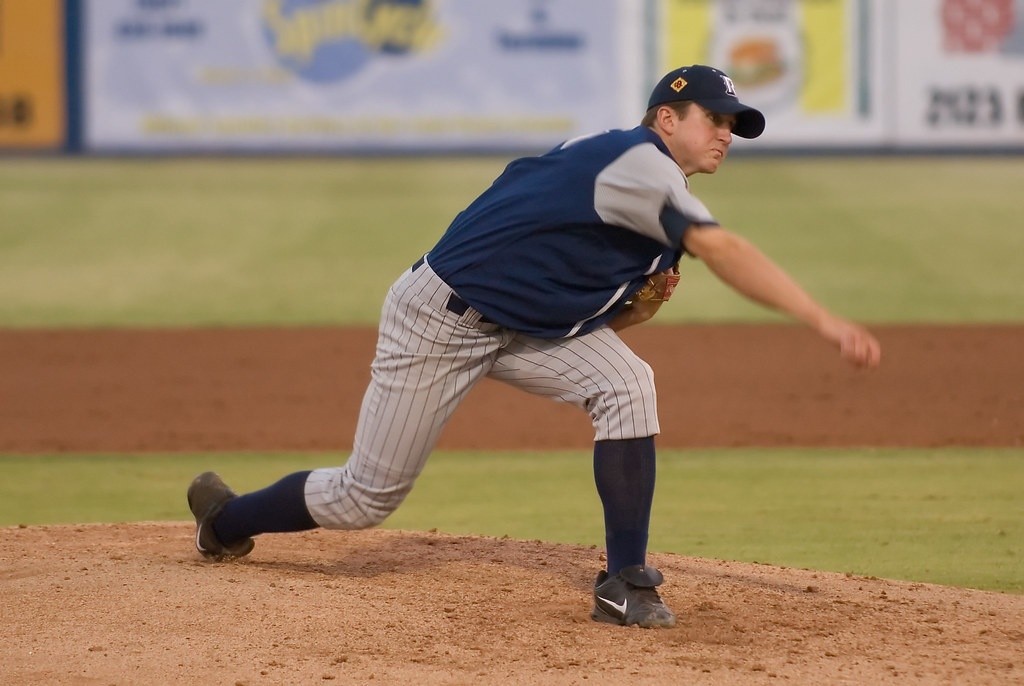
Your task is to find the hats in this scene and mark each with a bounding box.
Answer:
[647,64,765,140]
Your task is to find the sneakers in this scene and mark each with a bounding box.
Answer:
[187,472,256,564]
[591,565,677,629]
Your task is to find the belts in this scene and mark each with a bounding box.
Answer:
[412,251,495,324]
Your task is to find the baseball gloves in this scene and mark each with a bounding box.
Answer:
[627,260,682,303]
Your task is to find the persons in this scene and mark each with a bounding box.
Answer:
[190,65,884,627]
